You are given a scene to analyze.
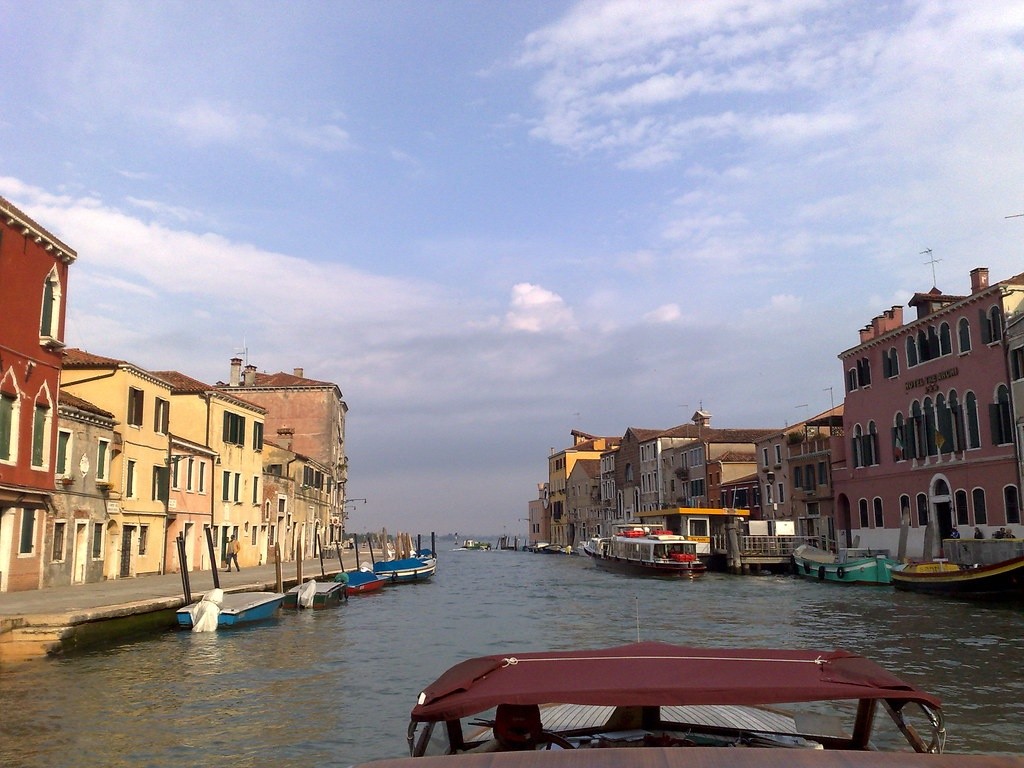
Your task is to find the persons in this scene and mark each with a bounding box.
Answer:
[492,703,543,752]
[992,527,1016,539]
[974,527,984,539]
[225,535,240,572]
[950,527,960,539]
[349,535,354,550]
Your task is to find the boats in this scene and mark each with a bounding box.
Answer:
[282,580,347,611]
[792,543,898,587]
[461,538,491,551]
[336,561,391,595]
[374,556,437,584]
[408,549,437,575]
[891,554,1024,603]
[527,541,590,557]
[583,524,707,580]
[176,587,286,633]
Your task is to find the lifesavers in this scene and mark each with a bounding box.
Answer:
[414,569,418,578]
[803,561,810,574]
[837,566,844,578]
[392,573,398,582]
[818,566,825,579]
[791,555,795,566]
